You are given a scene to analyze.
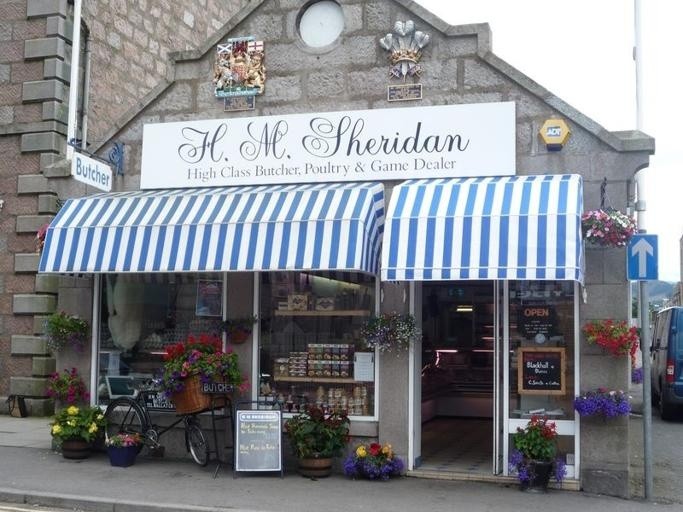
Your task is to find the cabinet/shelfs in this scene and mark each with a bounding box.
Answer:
[268,310,375,384]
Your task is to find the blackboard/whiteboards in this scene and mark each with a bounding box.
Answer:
[195,279,225,316]
[234,409,283,472]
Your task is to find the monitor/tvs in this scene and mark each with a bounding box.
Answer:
[105,374,136,399]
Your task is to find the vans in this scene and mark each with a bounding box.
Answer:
[650,303,683,422]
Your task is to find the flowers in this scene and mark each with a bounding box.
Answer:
[581,317,640,368]
[508,409,566,494]
[572,384,631,417]
[358,311,427,359]
[580,174,639,253]
[32,308,252,470]
[284,399,353,458]
[343,443,404,482]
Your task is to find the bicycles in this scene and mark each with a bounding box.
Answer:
[91,377,214,470]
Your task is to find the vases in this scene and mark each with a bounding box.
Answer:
[298,457,333,479]
[516,456,555,494]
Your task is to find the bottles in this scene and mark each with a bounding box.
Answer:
[258,379,369,416]
